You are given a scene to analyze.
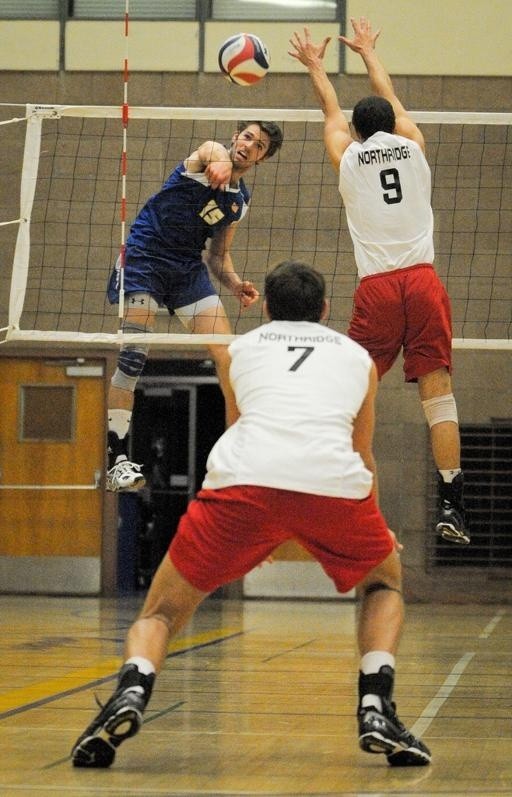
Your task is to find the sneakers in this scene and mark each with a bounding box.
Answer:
[69,681,148,768]
[357,707,431,765]
[104,452,146,495]
[434,498,472,546]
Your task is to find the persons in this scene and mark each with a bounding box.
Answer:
[70,258,434,768]
[287,15,472,547]
[105,119,283,493]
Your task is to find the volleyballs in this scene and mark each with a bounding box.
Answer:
[218,32,270,88]
